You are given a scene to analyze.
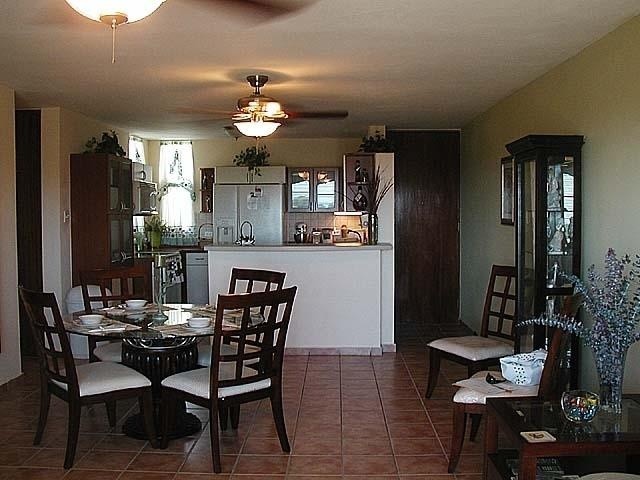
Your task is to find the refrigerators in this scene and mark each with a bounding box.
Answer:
[212,166,288,248]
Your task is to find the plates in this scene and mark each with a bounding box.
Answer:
[123,306,150,311]
[75,320,104,327]
[184,324,211,330]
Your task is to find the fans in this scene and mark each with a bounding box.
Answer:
[161,74,349,128]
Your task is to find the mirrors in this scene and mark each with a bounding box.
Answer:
[500,156,514,226]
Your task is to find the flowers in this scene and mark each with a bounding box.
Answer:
[516,248,640,413]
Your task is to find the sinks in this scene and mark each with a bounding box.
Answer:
[334,242,360,246]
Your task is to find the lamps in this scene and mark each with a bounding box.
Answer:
[64,0,168,64]
[232,121,282,139]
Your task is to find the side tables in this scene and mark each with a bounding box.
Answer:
[482,394,640,480]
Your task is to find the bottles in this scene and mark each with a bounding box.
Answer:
[352,187,368,210]
[143,238,147,250]
[354,160,369,184]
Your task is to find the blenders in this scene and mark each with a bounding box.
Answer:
[294,223,307,243]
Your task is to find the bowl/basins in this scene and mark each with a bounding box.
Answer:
[559,421,597,441]
[186,316,210,327]
[561,390,601,422]
[124,299,148,308]
[78,314,104,325]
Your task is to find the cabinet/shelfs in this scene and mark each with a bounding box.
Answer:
[342,152,375,212]
[287,167,340,212]
[71,215,134,270]
[186,252,208,305]
[70,153,133,215]
[504,134,584,354]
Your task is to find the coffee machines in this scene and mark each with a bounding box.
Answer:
[321,227,333,244]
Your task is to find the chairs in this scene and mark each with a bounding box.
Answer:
[160,286,297,474]
[448,293,586,473]
[76,269,130,362]
[425,264,516,399]
[18,285,161,470]
[198,267,285,431]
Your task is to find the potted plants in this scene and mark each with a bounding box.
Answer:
[359,130,396,152]
[144,215,168,248]
[334,162,394,245]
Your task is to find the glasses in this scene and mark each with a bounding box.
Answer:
[486,373,506,384]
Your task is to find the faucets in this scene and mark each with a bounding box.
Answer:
[240,221,252,240]
[198,223,213,242]
[347,229,362,243]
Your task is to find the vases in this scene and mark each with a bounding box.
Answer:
[585,345,629,414]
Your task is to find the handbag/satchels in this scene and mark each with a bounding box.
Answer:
[500,353,547,387]
